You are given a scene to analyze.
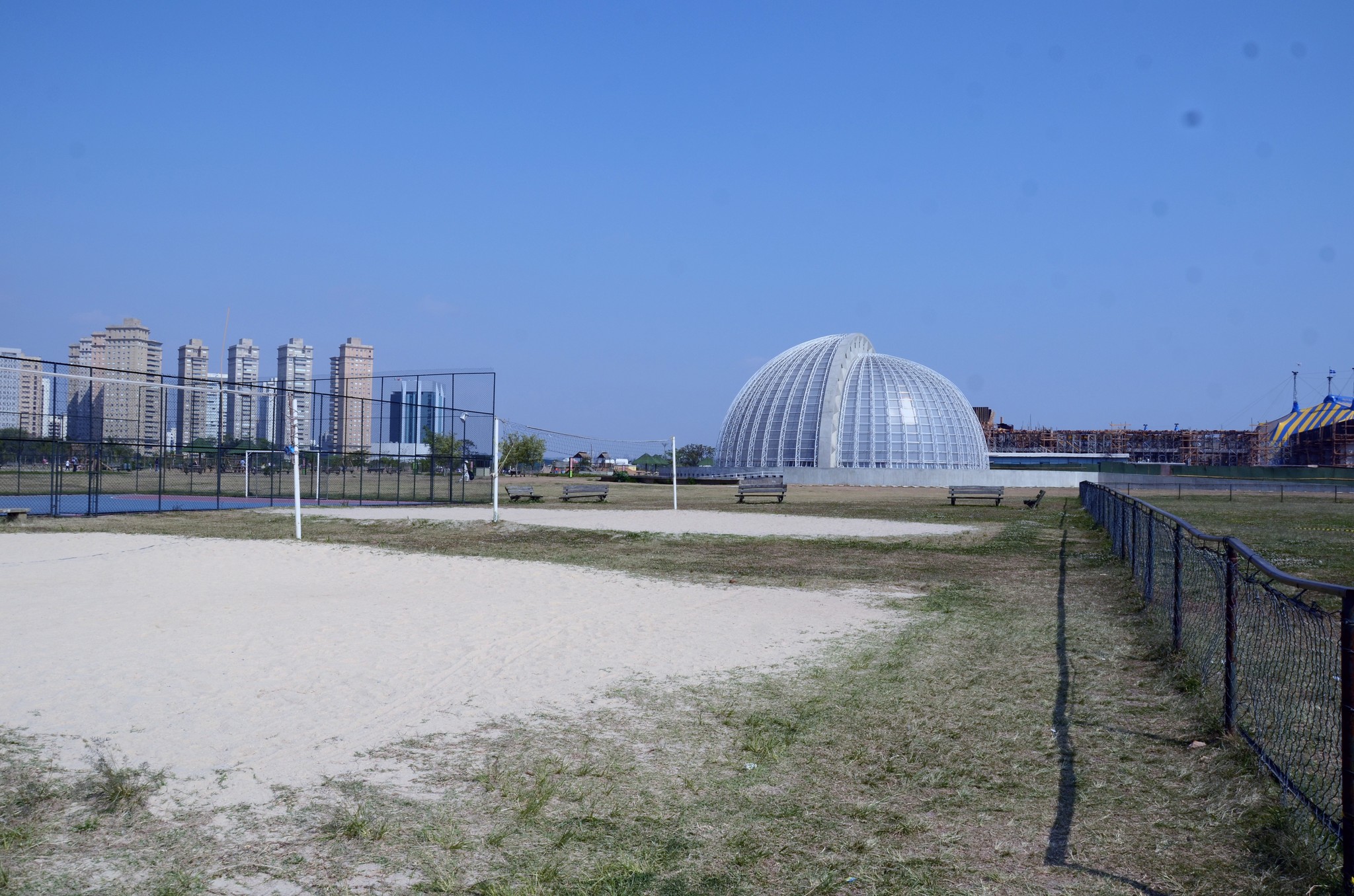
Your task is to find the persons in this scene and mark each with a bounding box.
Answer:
[43,455,470,483]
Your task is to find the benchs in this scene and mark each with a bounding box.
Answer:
[137,467,153,470]
[545,473,559,477]
[508,470,539,477]
[183,467,203,474]
[325,469,341,475]
[276,470,293,475]
[310,467,326,473]
[504,486,543,503]
[341,468,354,474]
[386,469,403,475]
[1023,489,1046,509]
[232,468,249,474]
[116,468,130,472]
[252,468,271,476]
[6,462,22,467]
[0,508,31,520]
[947,485,1004,507]
[366,467,385,473]
[735,485,787,502]
[167,466,182,471]
[559,485,609,501]
